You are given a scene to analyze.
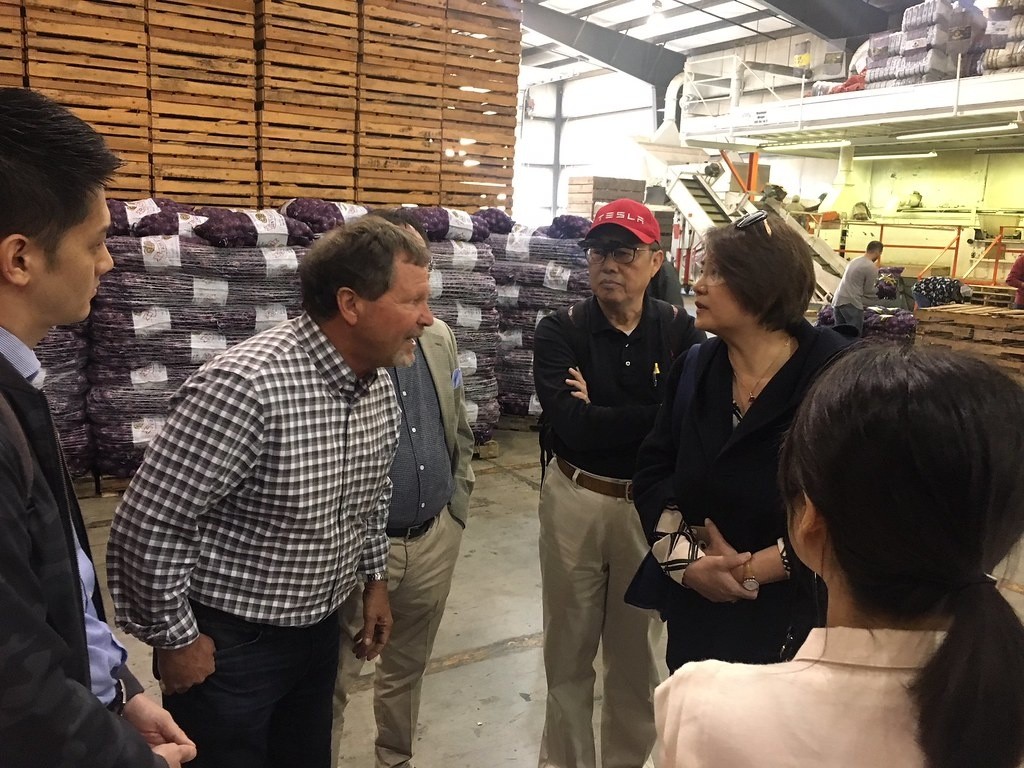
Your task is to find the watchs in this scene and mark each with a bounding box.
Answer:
[743,561,759,590]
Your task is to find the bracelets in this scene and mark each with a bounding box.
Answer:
[364,570,389,582]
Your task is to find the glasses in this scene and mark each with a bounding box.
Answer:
[735,208,773,238]
[583,247,654,264]
[693,261,730,286]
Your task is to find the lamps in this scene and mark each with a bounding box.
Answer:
[762,139,851,152]
[895,123,1020,139]
[852,149,938,161]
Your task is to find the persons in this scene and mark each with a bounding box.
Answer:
[532,198,707,768]
[331,213,475,767]
[1006,254,1024,309]
[0,87,198,768]
[624,214,853,678]
[652,345,1024,768]
[834,241,883,338]
[911,277,973,307]
[106,216,435,767]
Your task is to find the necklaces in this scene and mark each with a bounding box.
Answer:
[733,337,790,403]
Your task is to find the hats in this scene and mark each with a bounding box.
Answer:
[584,198,661,246]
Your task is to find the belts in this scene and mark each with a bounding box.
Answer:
[384,516,436,540]
[109,679,128,717]
[555,457,636,503]
